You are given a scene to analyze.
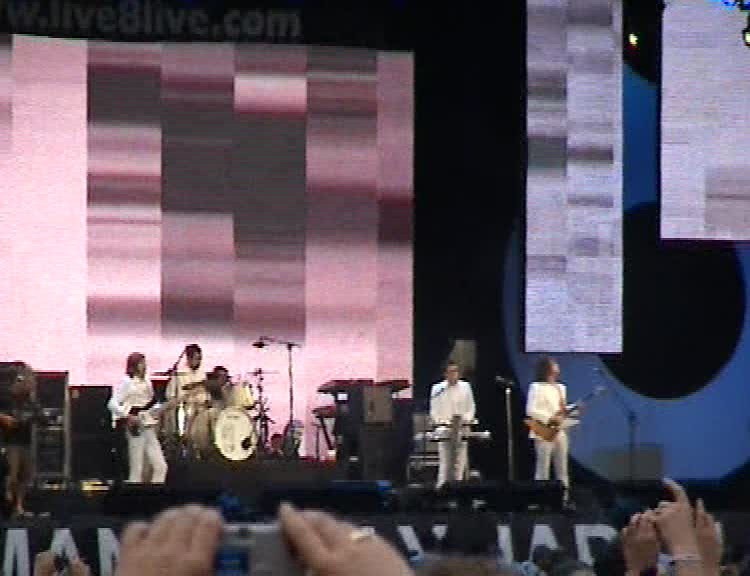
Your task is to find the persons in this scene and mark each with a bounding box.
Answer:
[164,344,211,438]
[106,352,169,485]
[429,360,477,490]
[618,476,726,576]
[33,551,90,576]
[524,356,582,507]
[209,366,235,406]
[0,369,38,518]
[112,499,414,575]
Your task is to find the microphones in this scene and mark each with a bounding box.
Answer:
[495,375,514,389]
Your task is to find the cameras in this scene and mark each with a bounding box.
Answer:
[211,524,307,575]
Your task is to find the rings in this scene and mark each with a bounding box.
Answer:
[350,525,375,543]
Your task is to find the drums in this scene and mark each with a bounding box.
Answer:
[220,382,254,411]
[187,405,257,467]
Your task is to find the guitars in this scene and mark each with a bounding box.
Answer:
[523,384,600,442]
[125,390,194,437]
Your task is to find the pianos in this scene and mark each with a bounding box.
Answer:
[412,427,493,481]
[429,419,479,427]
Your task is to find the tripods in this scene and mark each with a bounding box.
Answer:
[247,336,306,463]
